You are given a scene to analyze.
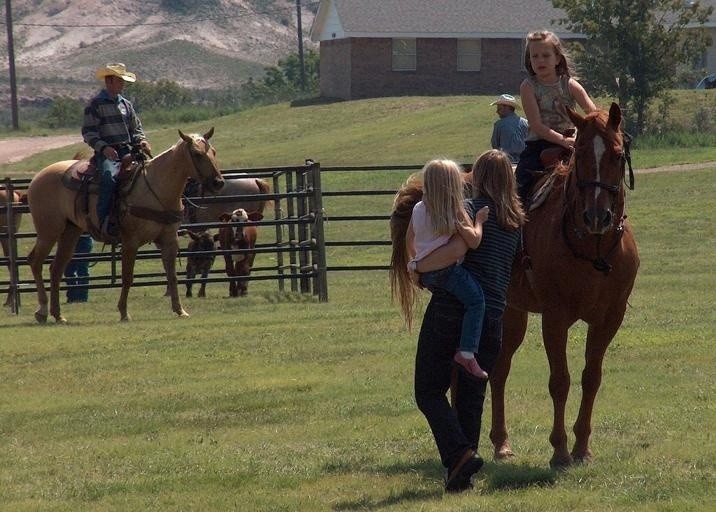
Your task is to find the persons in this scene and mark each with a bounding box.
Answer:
[414,149,530,493]
[405,158,490,380]
[80,62,150,237]
[512,29,599,210]
[488,94,529,173]
[62,232,92,303]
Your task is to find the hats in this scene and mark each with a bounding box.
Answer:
[489,94,522,112]
[95,63,136,83]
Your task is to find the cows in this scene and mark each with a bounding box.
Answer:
[184,227,221,298]
[219,208,267,297]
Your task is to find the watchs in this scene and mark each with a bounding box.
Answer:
[411,261,424,275]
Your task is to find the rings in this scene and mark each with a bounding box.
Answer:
[108,155,111,158]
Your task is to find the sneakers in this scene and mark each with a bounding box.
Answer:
[100,221,120,236]
[449,351,489,382]
[445,448,483,493]
[462,470,480,492]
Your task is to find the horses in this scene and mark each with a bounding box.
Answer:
[386,101,640,471]
[180,172,275,229]
[26,125,225,325]
[0,187,25,311]
